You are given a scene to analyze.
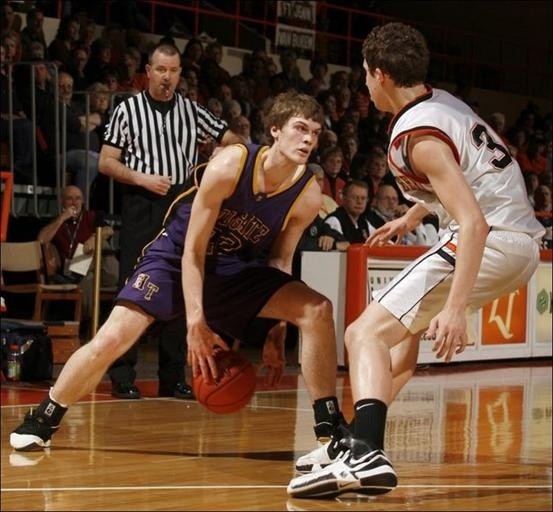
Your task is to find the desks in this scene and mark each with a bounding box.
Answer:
[296,250,553,369]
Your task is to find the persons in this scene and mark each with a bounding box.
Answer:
[34,75,98,209]
[99,44,246,401]
[9,95,348,452]
[307,49,390,200]
[323,181,394,252]
[365,185,426,246]
[0,0,50,180]
[285,23,543,497]
[177,30,300,153]
[38,186,119,346]
[489,97,553,251]
[44,13,154,117]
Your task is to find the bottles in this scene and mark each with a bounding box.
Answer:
[7,346,21,382]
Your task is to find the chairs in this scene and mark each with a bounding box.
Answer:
[0,240,83,339]
[41,241,119,323]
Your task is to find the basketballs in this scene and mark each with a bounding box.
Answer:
[193,347,257,414]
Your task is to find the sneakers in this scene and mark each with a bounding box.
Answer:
[296,424,353,473]
[285,438,398,499]
[11,410,62,452]
[313,414,349,449]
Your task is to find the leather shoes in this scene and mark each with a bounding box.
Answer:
[111,381,140,400]
[158,380,193,398]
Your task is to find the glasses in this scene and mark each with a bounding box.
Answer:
[377,196,398,202]
[535,191,552,196]
[346,195,370,202]
